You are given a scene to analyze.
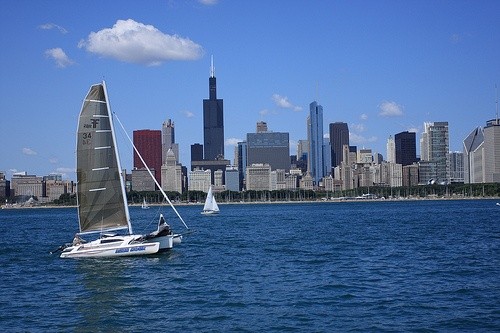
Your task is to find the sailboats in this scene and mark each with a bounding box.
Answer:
[201,187,220,217]
[142,198,149,209]
[59,81,190,259]
